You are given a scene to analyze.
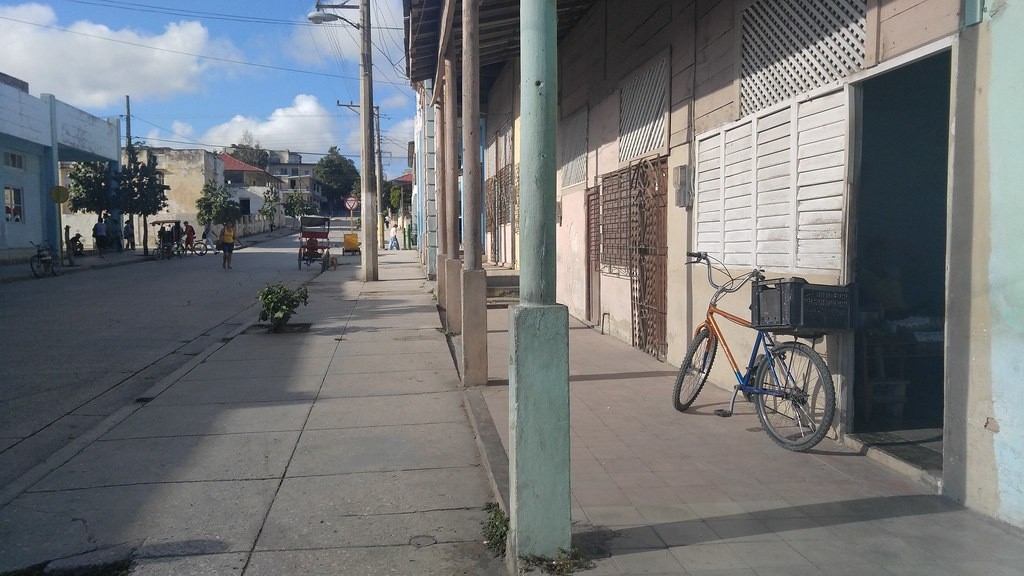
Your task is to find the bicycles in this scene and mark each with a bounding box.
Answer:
[672,250,836,454]
[28,240,62,279]
[172,234,208,256]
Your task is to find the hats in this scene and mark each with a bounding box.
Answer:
[76,233,82,237]
[207,218,212,221]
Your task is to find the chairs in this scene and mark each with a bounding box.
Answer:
[861,324,914,420]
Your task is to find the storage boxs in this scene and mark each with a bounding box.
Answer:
[750,278,858,332]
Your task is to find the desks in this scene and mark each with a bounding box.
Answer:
[872,337,945,415]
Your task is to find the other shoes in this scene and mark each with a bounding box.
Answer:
[223,263,226,268]
[200,252,204,255]
[215,252,219,255]
[228,266,232,269]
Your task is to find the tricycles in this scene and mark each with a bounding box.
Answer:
[151,219,185,260]
[297,215,335,271]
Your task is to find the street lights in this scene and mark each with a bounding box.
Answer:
[308,10,378,281]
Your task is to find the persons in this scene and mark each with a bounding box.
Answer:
[389,223,399,250]
[385,215,390,228]
[69,234,83,256]
[93,213,135,258]
[200,219,219,255]
[219,219,243,269]
[160,221,194,257]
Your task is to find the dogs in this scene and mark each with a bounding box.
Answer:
[330,256,337,268]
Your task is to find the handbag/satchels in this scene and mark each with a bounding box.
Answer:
[216,240,223,251]
[202,231,206,239]
[93,224,97,238]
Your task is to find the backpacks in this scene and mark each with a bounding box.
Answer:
[190,226,195,236]
